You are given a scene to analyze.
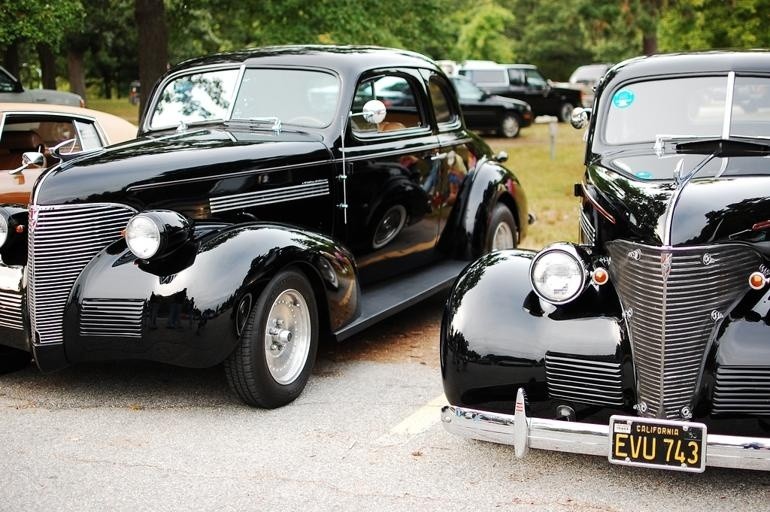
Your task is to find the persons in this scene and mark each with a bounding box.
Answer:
[396,145,479,210]
